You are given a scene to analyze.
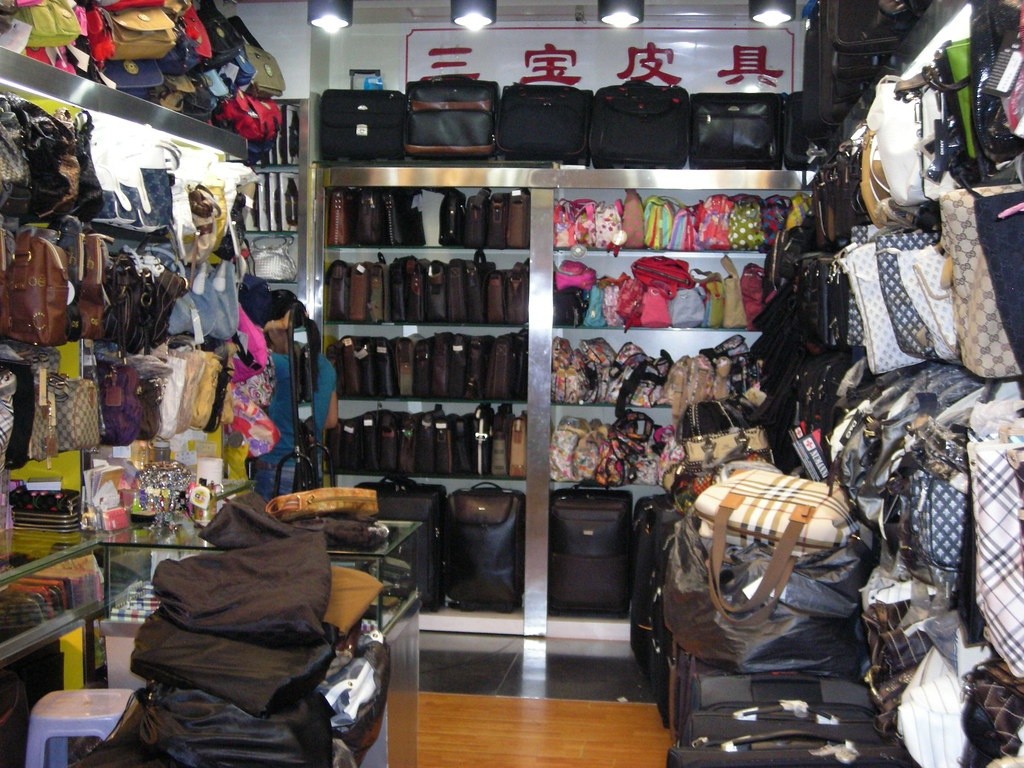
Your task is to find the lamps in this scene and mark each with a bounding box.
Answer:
[748,0,796,25]
[451,0,497,28]
[306,0,354,31]
[597,0,645,29]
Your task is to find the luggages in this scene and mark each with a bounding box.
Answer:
[352,478,446,617]
[548,480,632,618]
[446,481,527,614]
[629,492,921,767]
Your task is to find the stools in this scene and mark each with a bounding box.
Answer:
[25,687,134,768]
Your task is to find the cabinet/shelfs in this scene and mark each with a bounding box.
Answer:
[0,519,423,768]
[314,167,825,641]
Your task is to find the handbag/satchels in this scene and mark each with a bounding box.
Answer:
[0,1,1024,477]
[694,466,864,631]
